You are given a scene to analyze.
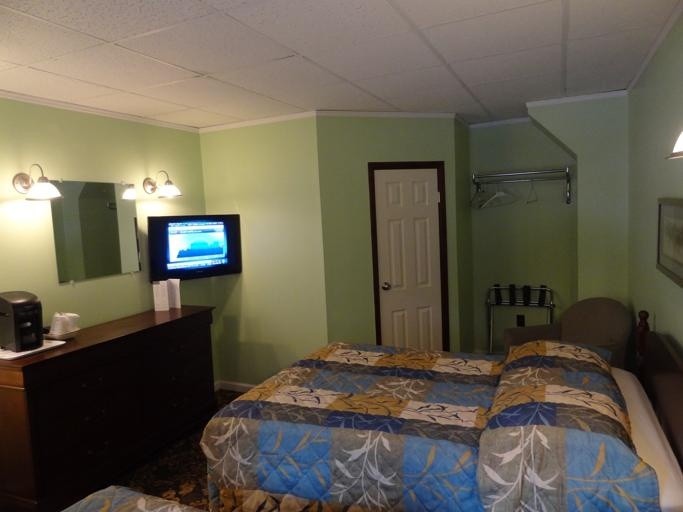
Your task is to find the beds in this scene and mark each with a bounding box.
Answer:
[200,311,683,512]
[60,476,213,511]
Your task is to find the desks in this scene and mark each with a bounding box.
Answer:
[0,304,216,510]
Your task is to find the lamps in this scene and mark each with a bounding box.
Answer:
[13,163,62,205]
[121,185,135,201]
[663,131,683,161]
[144,170,182,198]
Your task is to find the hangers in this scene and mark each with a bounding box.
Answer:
[469,181,491,204]
[478,185,518,209]
[526,177,539,207]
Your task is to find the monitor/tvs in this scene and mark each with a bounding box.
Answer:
[148,215,241,285]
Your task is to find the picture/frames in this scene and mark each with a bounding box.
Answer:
[656,198,683,287]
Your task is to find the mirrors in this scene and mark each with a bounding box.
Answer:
[48,180,145,285]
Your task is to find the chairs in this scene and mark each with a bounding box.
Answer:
[503,296,634,372]
